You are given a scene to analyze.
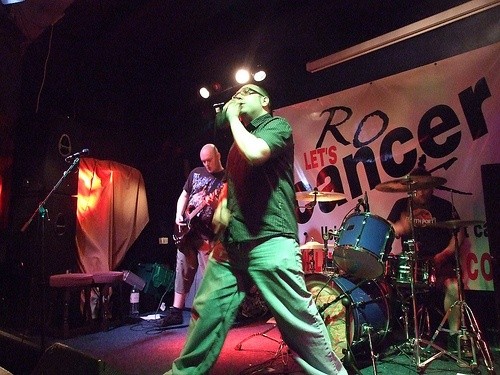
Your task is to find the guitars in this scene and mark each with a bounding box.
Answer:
[171,184,224,246]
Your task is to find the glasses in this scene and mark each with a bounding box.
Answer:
[232,87,265,100]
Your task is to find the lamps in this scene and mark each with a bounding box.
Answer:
[195,79,222,101]
[233,57,251,85]
[251,59,268,81]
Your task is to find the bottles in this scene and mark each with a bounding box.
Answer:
[129,284,139,317]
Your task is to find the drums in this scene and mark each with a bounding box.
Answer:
[303,272,391,364]
[332,211,396,281]
[386,254,437,287]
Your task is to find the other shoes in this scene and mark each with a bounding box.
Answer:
[154,306,184,327]
[449,333,473,359]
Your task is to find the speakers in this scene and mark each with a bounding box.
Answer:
[0,326,106,375]
[0,194,77,334]
[11,105,79,196]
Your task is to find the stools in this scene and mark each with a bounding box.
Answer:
[48,272,94,343]
[92,271,124,333]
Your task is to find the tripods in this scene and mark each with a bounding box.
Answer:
[376,184,498,375]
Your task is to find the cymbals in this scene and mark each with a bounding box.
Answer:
[299,240,336,250]
[374,174,447,193]
[426,218,487,230]
[295,190,347,202]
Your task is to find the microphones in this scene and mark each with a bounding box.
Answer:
[65,149,89,161]
[363,191,370,213]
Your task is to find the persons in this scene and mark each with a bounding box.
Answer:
[163,83,347,375]
[386,168,473,360]
[154,144,225,327]
